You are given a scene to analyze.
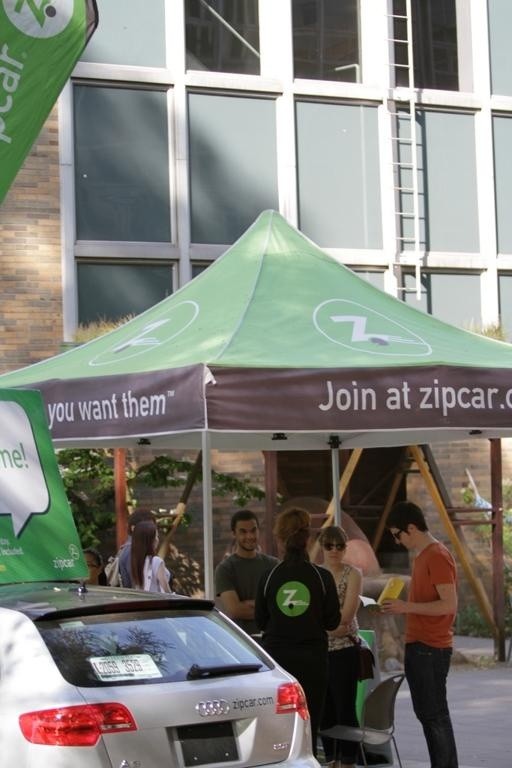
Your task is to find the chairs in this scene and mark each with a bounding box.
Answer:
[317,672,406,767]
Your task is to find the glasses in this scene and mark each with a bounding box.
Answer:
[394,530,403,540]
[322,543,345,551]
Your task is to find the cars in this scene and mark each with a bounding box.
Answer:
[0,582,325,768]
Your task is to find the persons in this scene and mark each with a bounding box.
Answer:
[249,507,341,759]
[374,498,459,767]
[313,525,367,767]
[80,545,114,588]
[212,509,284,642]
[128,521,173,594]
[117,508,158,588]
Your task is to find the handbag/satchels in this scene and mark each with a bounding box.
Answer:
[104,556,120,587]
[357,635,376,682]
